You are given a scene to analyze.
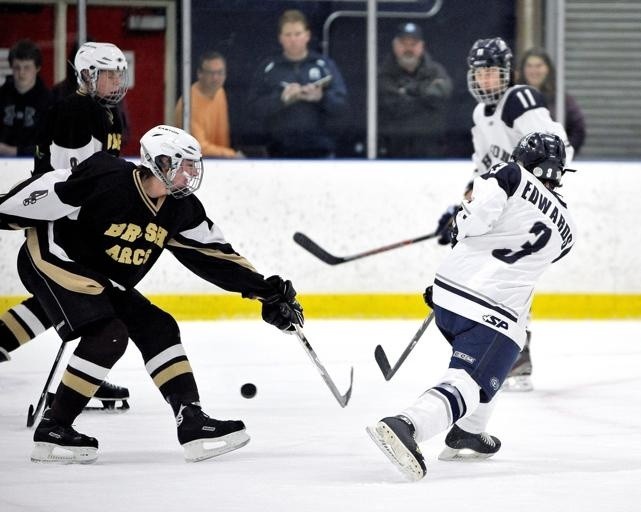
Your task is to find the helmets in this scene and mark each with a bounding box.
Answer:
[139,124,204,200]
[466,36,514,106]
[73,41,129,108]
[510,130,566,184]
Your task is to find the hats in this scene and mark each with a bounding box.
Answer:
[392,21,427,40]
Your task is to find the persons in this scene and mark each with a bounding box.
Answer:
[521,48,585,154]
[450,36,575,375]
[1,41,129,412]
[0,40,47,159]
[174,50,245,159]
[247,11,352,158]
[377,131,577,479]
[377,23,460,158]
[1,124,304,464]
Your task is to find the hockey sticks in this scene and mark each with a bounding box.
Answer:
[373,310,435,381]
[285,305,354,409]
[23,337,67,429]
[292,226,442,266]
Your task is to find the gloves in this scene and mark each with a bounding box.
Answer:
[257,274,305,336]
[434,204,462,246]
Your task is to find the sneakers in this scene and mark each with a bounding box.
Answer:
[363,414,427,483]
[93,380,131,408]
[437,425,503,464]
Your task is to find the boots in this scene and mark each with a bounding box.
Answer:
[30,392,99,466]
[500,326,534,395]
[1,348,10,363]
[172,401,253,465]
